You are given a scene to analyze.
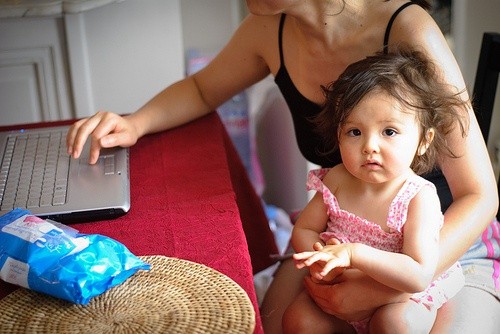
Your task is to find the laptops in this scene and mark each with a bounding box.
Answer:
[0,126,130,222]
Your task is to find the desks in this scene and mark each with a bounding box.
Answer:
[1,111,284,334]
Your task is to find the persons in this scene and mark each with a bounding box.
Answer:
[279,46,470,334]
[63,0,499,334]
